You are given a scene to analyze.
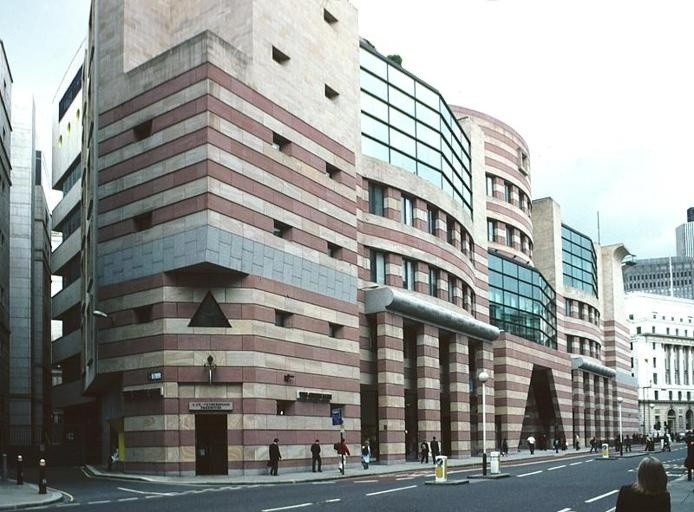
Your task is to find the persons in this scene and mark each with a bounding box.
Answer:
[269,438,283,476]
[311,440,323,472]
[502,431,693,481]
[616,456,669,512]
[421,440,429,463]
[361,441,371,469]
[336,439,350,472]
[431,436,441,464]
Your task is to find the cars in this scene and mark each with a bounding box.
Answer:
[676,432,686,443]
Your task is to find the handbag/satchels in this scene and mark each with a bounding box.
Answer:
[362,447,368,455]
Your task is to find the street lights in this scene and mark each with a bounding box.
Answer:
[616,396,624,456]
[477,370,490,475]
